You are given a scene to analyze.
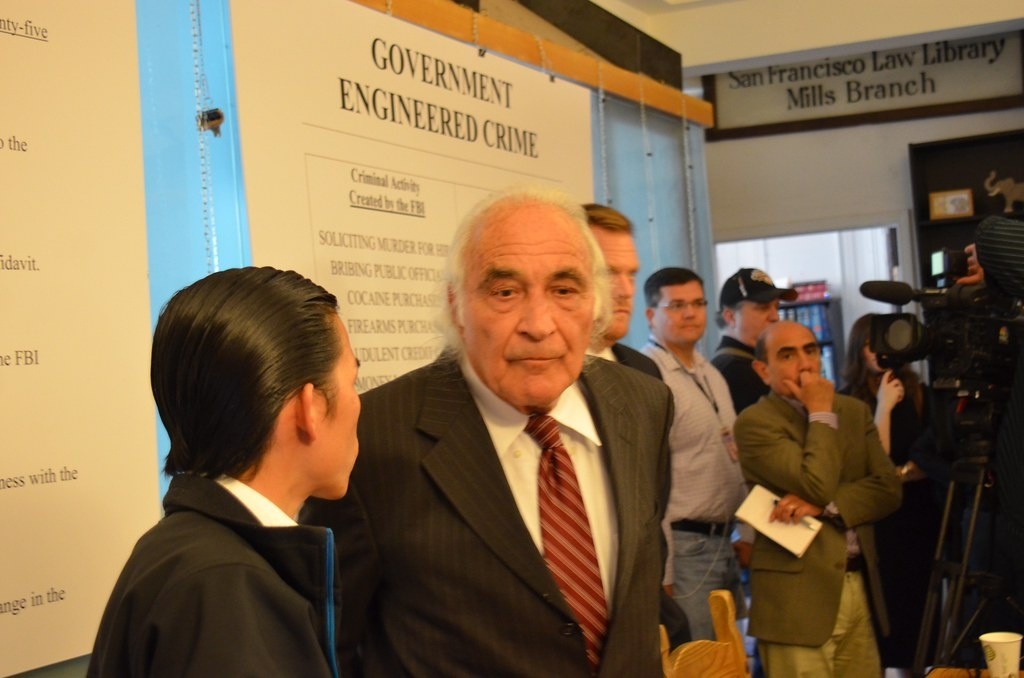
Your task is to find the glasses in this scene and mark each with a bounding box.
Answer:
[655,299,708,314]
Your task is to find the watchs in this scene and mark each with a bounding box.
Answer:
[826,500,839,519]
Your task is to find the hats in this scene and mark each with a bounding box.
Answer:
[719,268,798,304]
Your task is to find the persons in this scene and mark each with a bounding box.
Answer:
[636,216,1024,678]
[85,266,360,678]
[293,195,676,678]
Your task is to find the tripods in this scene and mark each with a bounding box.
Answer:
[914,403,1024,678]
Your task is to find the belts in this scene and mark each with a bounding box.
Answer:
[670,519,733,536]
[847,554,865,571]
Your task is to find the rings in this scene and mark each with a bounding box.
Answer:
[785,502,796,509]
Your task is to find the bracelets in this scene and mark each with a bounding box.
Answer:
[900,466,913,481]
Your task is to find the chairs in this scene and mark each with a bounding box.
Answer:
[659,589,754,678]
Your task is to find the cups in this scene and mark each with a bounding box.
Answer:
[978,632,1023,678]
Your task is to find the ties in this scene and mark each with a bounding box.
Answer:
[522,414,612,678]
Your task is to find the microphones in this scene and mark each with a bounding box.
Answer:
[860,281,947,306]
